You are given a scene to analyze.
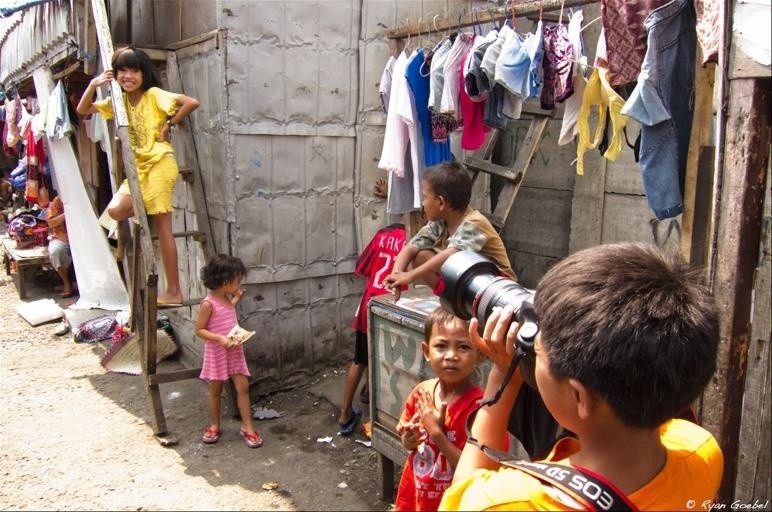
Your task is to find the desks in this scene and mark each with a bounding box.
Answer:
[2,238,50,300]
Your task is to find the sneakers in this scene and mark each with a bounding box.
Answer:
[360,384,369,404]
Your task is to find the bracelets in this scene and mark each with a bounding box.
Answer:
[167,119,176,130]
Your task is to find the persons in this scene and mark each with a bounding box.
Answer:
[439,238,725,511]
[46,195,76,298]
[374,177,388,199]
[338,209,411,436]
[393,305,486,512]
[76,46,200,308]
[195,253,263,449]
[382,160,518,307]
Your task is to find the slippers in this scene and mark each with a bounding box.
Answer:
[340,407,362,433]
[240,430,264,448]
[202,425,222,443]
[157,298,184,307]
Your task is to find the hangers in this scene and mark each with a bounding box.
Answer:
[385,0,610,78]
